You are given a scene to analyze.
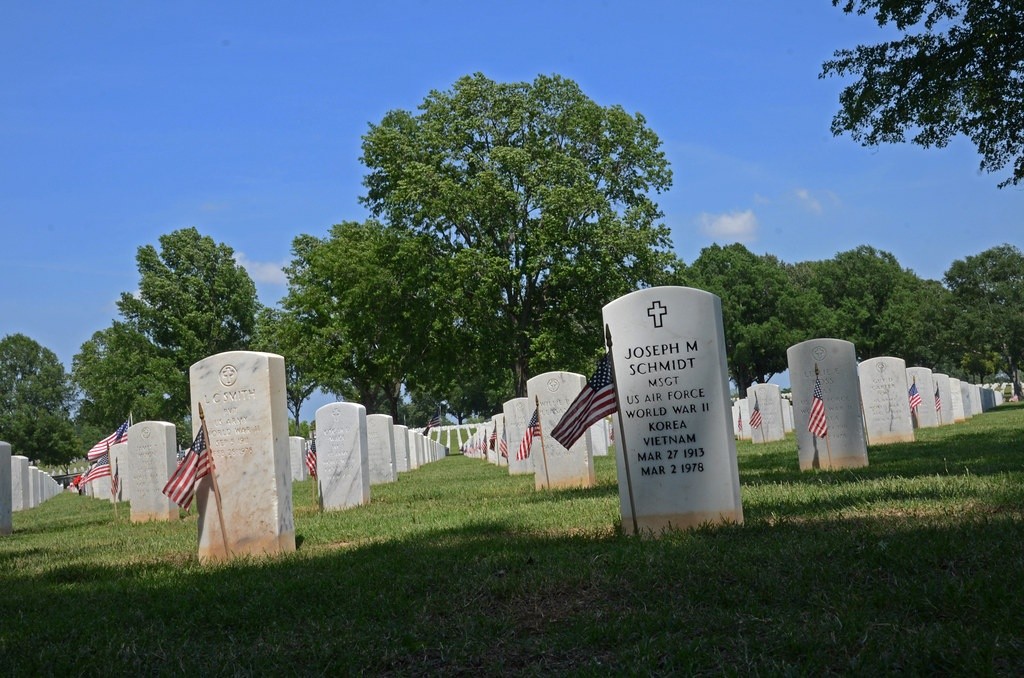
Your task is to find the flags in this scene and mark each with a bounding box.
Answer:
[489,424,497,452]
[749,403,762,430]
[550,353,617,450]
[499,427,508,459]
[84,455,111,483]
[162,424,214,514]
[909,384,922,415]
[737,409,742,432]
[427,406,440,428]
[516,409,541,461]
[935,388,941,411]
[305,430,317,481]
[808,379,827,438]
[88,417,128,460]
[481,434,487,454]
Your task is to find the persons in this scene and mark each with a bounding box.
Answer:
[74,475,83,495]
[1015,384,1024,399]
